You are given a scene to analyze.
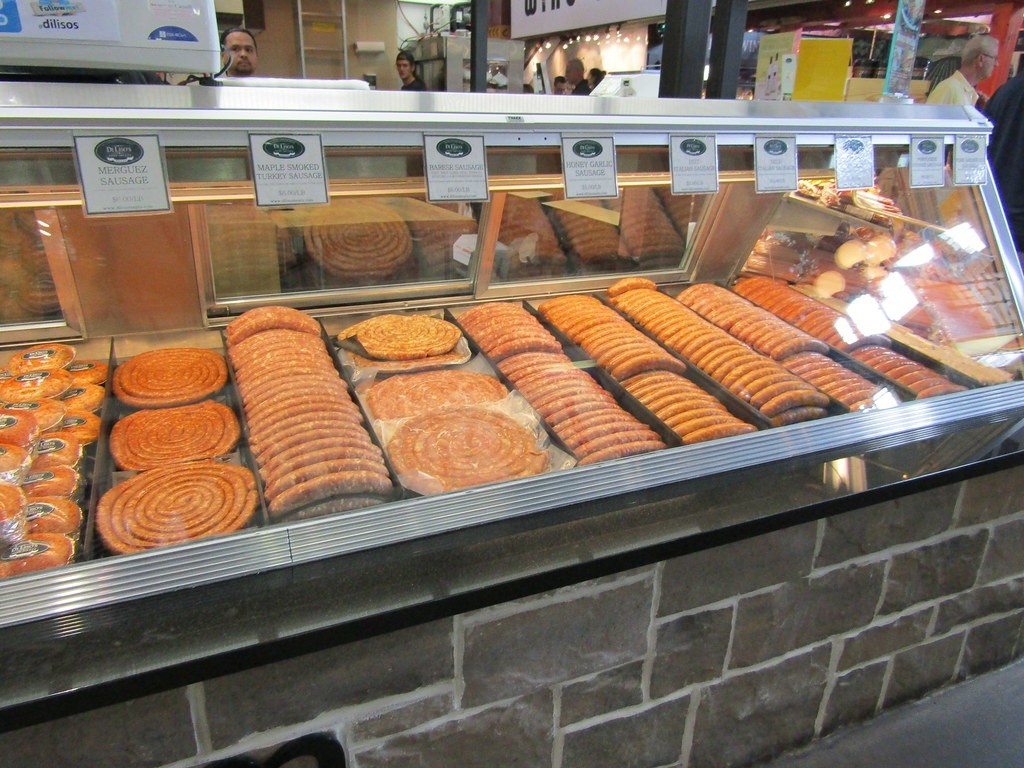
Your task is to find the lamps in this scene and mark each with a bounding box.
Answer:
[545,36,552,49]
[604,25,611,40]
[585,27,592,42]
[593,27,601,41]
[536,37,544,53]
[568,32,573,45]
[562,34,568,50]
[616,23,622,38]
[576,30,581,42]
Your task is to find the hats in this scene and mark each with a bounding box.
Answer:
[397,52,414,63]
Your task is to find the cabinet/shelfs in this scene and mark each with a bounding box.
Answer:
[209,0,246,78]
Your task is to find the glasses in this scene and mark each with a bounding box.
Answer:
[976,53,998,62]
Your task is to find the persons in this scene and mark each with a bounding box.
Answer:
[554,58,606,96]
[220,28,258,77]
[36,68,168,85]
[926,34,1000,109]
[982,71,1024,257]
[396,50,427,91]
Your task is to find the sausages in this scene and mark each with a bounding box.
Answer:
[97,347,259,557]
[225,305,393,521]
[299,180,708,282]
[537,277,972,445]
[335,315,548,497]
[453,301,668,468]
